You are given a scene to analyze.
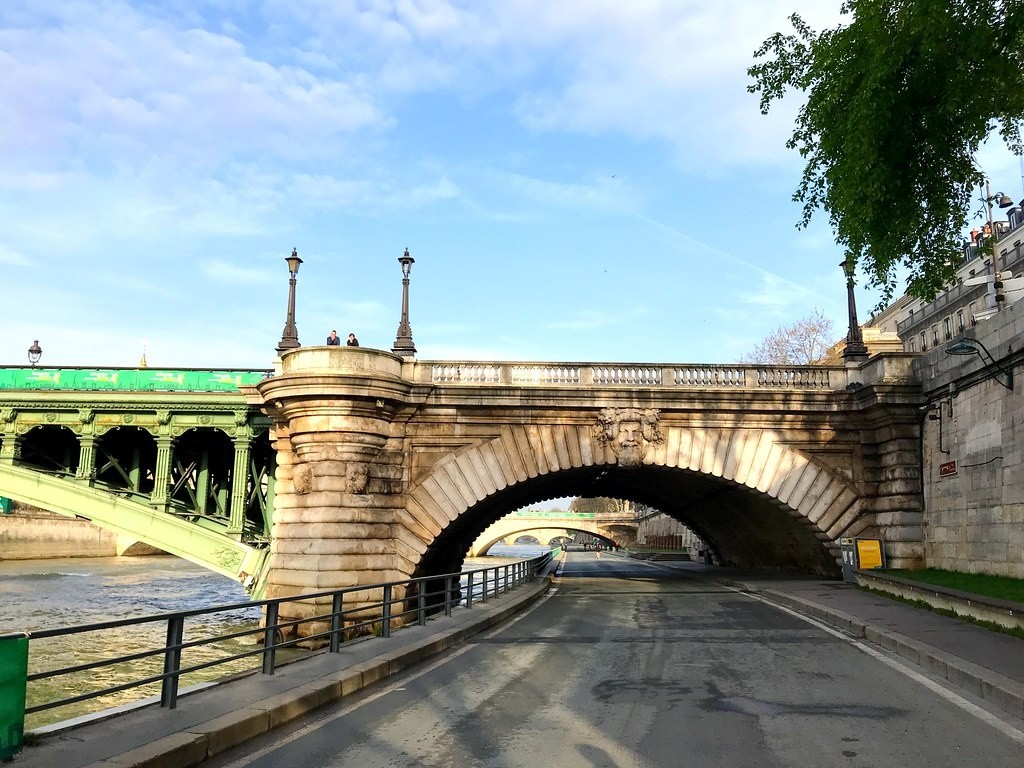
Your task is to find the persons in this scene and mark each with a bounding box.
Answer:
[583,542,602,559]
[327,330,340,345]
[347,333,360,347]
